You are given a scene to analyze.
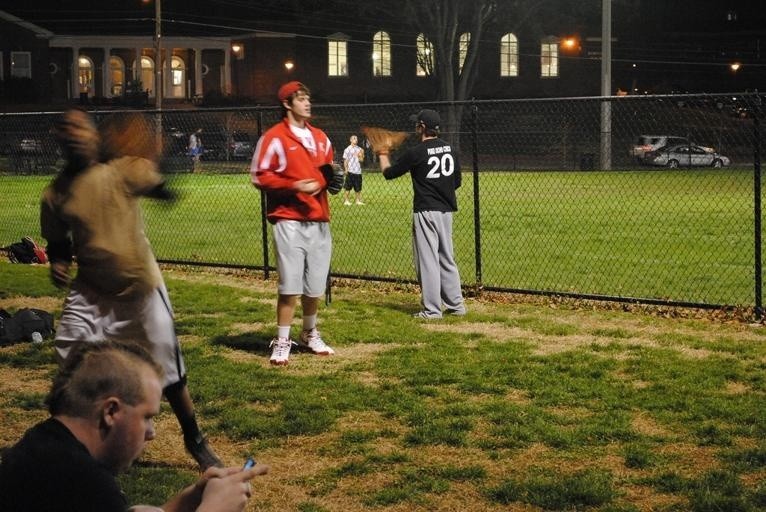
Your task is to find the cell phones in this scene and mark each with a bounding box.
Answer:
[243,458,257,471]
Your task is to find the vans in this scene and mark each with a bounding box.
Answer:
[176,129,253,161]
[558,125,646,170]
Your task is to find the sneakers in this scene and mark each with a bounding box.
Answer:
[182,430,222,471]
[268,337,292,366]
[299,328,335,355]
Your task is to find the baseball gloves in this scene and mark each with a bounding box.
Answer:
[363,126,409,155]
[105,114,153,161]
[320,162,343,195]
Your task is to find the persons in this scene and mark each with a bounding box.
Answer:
[38,107,221,474]
[376,108,466,322]
[341,134,365,205]
[0,334,267,511]
[186,125,205,172]
[250,81,343,367]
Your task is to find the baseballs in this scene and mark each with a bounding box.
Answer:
[305,180,320,192]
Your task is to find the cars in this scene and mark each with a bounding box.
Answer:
[658,88,766,119]
[0,116,66,157]
[163,127,188,139]
[629,135,716,162]
[644,144,730,169]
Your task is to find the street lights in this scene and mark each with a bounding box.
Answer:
[232,42,241,99]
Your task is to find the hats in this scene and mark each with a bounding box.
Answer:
[409,109,441,129]
[277,81,303,102]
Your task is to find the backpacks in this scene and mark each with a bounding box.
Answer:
[0,307,55,347]
